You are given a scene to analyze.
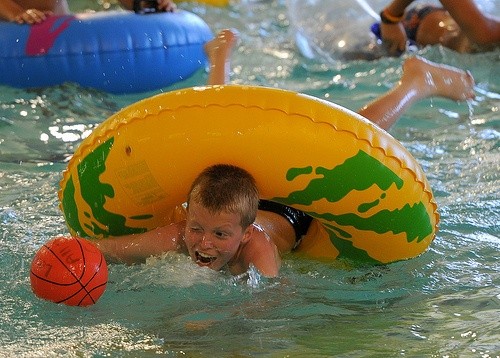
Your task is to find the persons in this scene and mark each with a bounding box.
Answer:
[57,27,475,333]
[0,0,176,26]
[370,0,499,60]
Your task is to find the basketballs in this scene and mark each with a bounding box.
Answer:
[30,236,108,308]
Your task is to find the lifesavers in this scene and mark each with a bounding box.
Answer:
[56,85,440,269]
[0,7,216,94]
